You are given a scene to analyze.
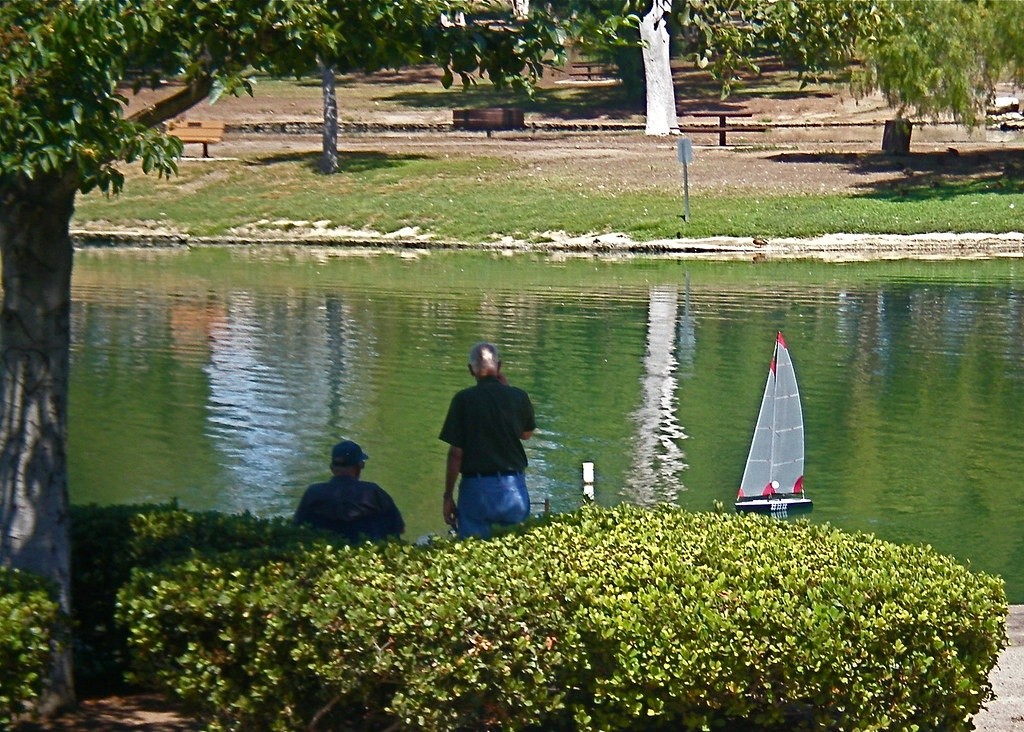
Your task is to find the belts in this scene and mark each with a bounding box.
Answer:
[461,470,522,478]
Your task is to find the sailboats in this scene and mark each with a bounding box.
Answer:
[735,328,813,513]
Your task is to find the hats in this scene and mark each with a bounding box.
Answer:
[333,440,368,466]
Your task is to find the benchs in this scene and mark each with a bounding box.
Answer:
[568,64,612,81]
[451,109,525,134]
[164,121,225,158]
[678,113,766,145]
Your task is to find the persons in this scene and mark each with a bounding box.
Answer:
[293,441,405,543]
[438,343,537,540]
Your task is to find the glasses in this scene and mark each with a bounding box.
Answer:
[358,461,365,468]
